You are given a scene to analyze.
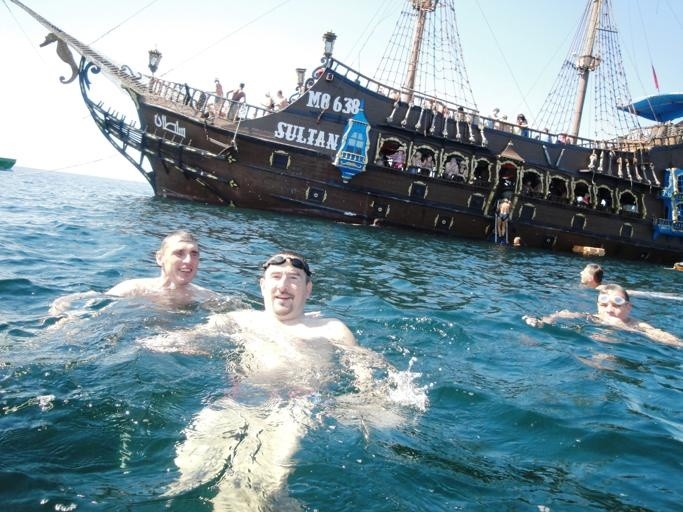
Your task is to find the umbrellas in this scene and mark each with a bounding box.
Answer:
[618,94,683,123]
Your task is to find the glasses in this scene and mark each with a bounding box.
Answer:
[598,295,629,305]
[266,256,304,269]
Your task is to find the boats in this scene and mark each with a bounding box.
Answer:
[0,157,16,171]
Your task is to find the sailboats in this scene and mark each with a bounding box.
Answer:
[1,1,682,266]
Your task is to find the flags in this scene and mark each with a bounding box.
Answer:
[651,64,659,91]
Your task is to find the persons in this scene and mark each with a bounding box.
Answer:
[226,83,246,122]
[521,284,683,350]
[214,81,223,110]
[512,236,522,247]
[51,231,253,320]
[276,90,289,110]
[580,263,683,301]
[134,254,372,512]
[376,106,608,210]
[496,198,512,241]
[261,93,274,115]
[663,262,683,272]
